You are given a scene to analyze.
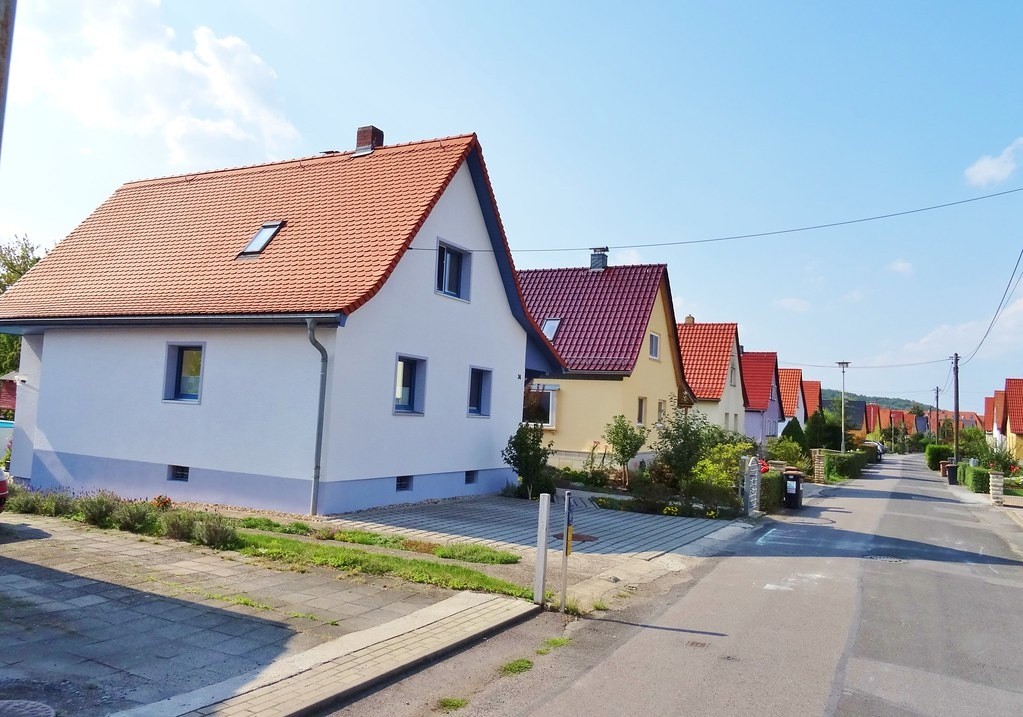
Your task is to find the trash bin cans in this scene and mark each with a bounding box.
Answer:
[945,464,960,485]
[783,466,806,510]
[939,461,951,478]
